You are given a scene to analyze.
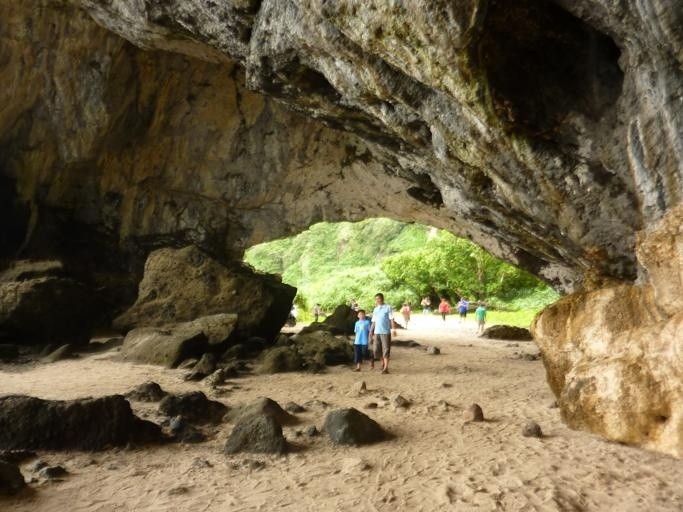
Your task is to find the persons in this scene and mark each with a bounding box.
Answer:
[474,300,486,333]
[399,301,411,330]
[370,292,397,374]
[350,298,355,310]
[314,302,322,322]
[353,309,374,372]
[354,303,358,312]
[290,304,298,327]
[437,297,450,322]
[455,297,469,323]
[420,295,431,316]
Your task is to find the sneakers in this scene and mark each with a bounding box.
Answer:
[380,366,389,374]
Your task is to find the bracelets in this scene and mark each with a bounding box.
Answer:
[390,328,396,332]
